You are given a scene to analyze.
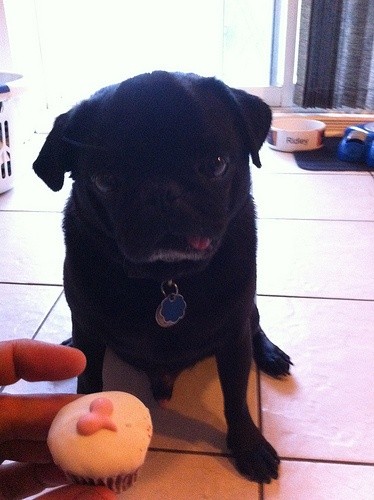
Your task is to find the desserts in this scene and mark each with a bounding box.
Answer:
[47,391,153,494]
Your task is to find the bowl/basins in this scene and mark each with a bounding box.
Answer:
[265,118,326,152]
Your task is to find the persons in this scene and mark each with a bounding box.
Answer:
[0,339,118,500]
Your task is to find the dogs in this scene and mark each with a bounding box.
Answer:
[32,68,294,485]
